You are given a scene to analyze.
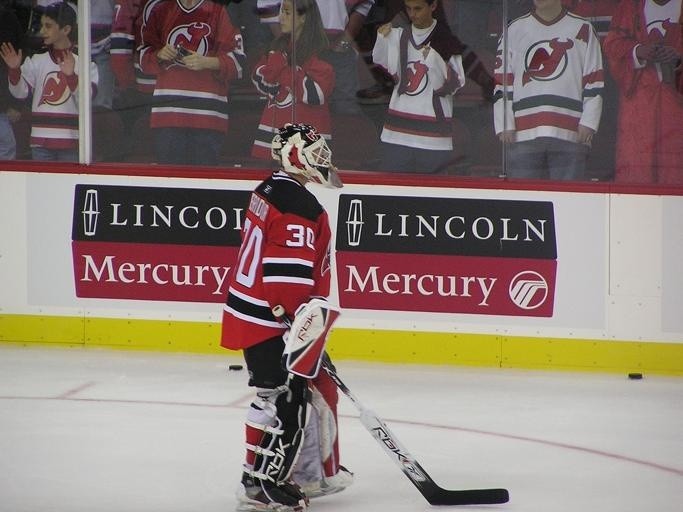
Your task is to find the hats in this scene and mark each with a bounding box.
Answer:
[33,2,76,27]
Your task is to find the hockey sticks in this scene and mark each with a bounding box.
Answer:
[272,306,508,505]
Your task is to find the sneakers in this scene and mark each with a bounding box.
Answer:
[236,473,273,506]
[355,81,395,97]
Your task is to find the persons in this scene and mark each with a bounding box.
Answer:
[135,0,336,167]
[0,0,146,163]
[370,1,460,175]
[602,1,683,183]
[492,1,604,179]
[218,125,354,512]
[316,1,376,145]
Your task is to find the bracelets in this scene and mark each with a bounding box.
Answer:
[335,40,351,48]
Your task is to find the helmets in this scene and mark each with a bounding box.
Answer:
[271,123,343,188]
